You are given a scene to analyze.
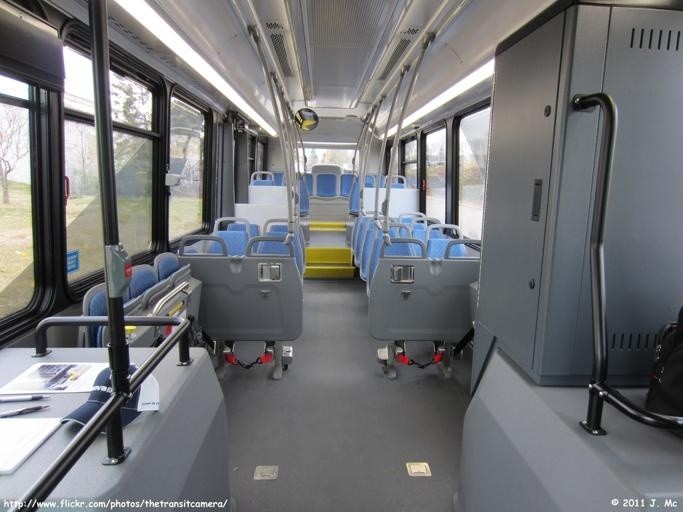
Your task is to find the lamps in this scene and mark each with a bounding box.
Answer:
[378,51,496,139]
[114,0,280,139]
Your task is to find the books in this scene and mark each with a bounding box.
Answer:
[0,417,65,477]
[0,357,134,394]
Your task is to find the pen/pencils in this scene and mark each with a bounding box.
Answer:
[0,395,50,403]
[0,405,49,418]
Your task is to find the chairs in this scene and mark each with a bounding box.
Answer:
[75,164,482,382]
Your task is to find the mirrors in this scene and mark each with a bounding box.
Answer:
[295,108,319,131]
